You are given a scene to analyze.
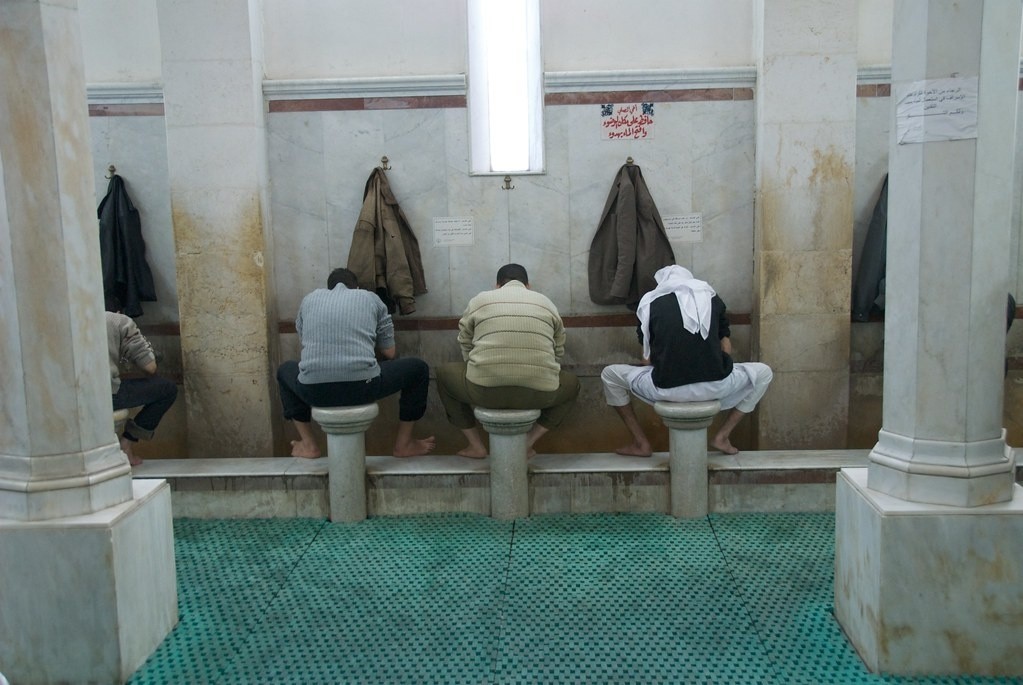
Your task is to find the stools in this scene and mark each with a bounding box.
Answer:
[475,407,543,523]
[655,400,719,518]
[311,402,380,523]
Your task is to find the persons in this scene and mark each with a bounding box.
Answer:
[104,292,177,465]
[276,268,436,459]
[438,263,581,458]
[601,265,773,457]
